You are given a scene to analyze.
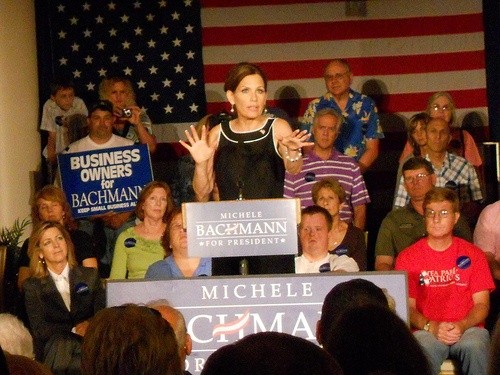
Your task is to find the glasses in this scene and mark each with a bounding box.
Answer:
[431,103,451,111]
[326,72,348,81]
[426,209,454,219]
[404,173,431,183]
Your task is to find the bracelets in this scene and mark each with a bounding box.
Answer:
[285,147,302,162]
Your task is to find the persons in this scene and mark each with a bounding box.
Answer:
[473,198,500,336]
[40,83,88,184]
[296,177,368,271]
[109,181,174,279]
[0,312,52,374]
[81,303,192,374]
[143,206,211,280]
[283,108,371,230]
[394,113,433,196]
[395,186,493,375]
[199,278,434,374]
[179,62,314,201]
[98,76,152,143]
[16,184,98,293]
[53,99,159,265]
[375,156,485,272]
[398,92,485,193]
[394,118,484,206]
[294,205,359,273]
[302,59,385,173]
[23,221,106,375]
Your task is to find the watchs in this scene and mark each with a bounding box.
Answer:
[423,320,431,332]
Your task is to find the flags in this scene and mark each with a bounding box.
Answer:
[34,0,489,156]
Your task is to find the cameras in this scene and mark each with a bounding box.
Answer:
[123,108,131,117]
